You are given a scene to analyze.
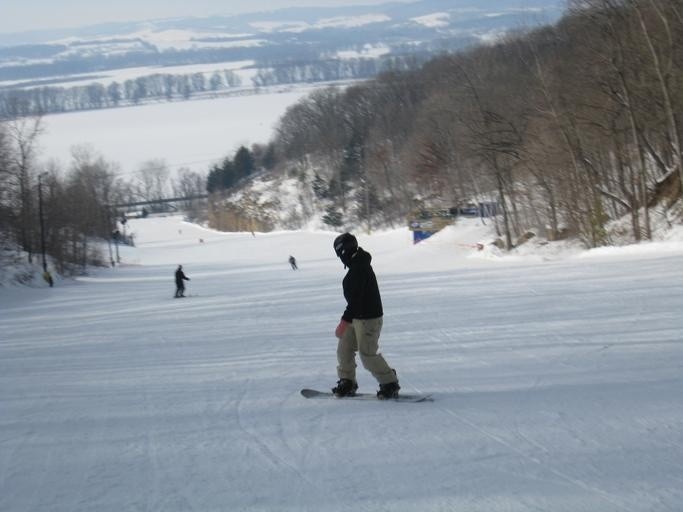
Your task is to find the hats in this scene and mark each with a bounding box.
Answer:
[333,232,358,257]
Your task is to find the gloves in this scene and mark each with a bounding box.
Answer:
[335,320,347,337]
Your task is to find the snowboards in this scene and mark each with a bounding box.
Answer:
[301,389,433,402]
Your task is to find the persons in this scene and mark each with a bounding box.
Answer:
[172,264,189,297]
[332,232,399,400]
[288,255,297,271]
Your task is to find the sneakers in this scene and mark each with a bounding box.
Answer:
[376,382,401,399]
[332,379,358,395]
